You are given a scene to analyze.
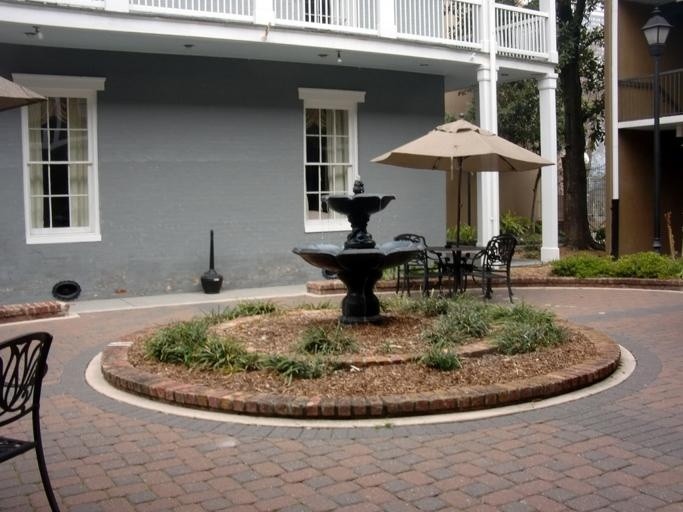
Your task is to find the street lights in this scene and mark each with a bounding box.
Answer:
[641,7,673,255]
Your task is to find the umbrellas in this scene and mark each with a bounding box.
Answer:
[0,74,47,116]
[367,111,559,246]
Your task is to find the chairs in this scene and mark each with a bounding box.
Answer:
[0,332,60,512]
[393,234,517,304]
